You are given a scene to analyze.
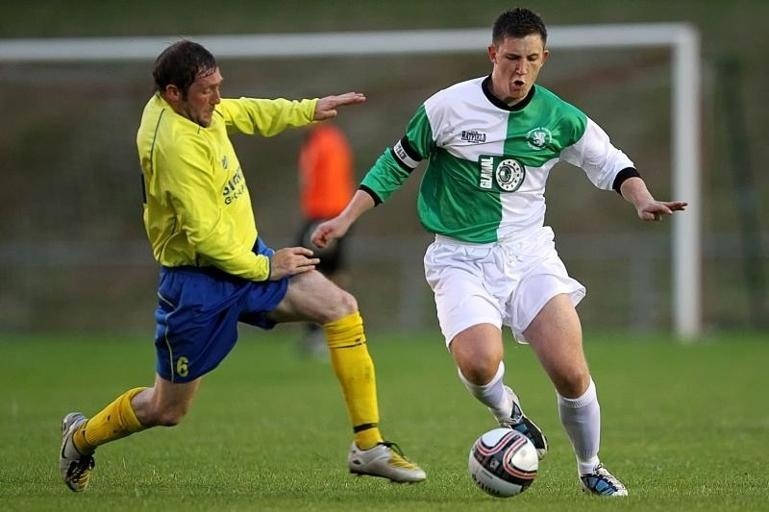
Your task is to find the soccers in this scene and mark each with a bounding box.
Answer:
[469,426,538,496]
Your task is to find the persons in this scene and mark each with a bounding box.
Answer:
[309,6,689,495]
[59,39,426,493]
[285,91,361,356]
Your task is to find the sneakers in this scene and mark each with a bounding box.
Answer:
[56,410,96,494]
[344,441,429,486]
[486,383,550,462]
[579,465,631,499]
